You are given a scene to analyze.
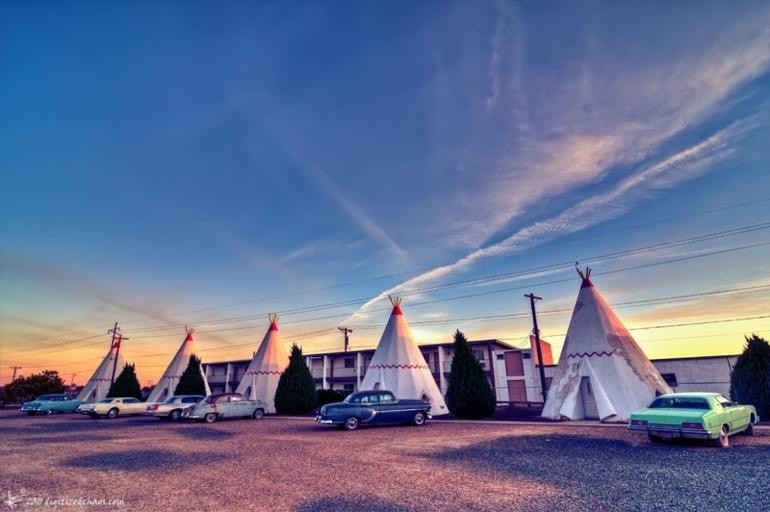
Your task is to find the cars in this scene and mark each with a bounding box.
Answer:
[629,391,761,451]
[180,393,268,423]
[20,393,86,414]
[75,397,152,420]
[145,395,206,421]
[315,389,433,430]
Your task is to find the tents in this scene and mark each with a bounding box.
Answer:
[234,314,291,413]
[541,266,675,424]
[76,342,127,402]
[360,295,450,420]
[145,325,211,413]
